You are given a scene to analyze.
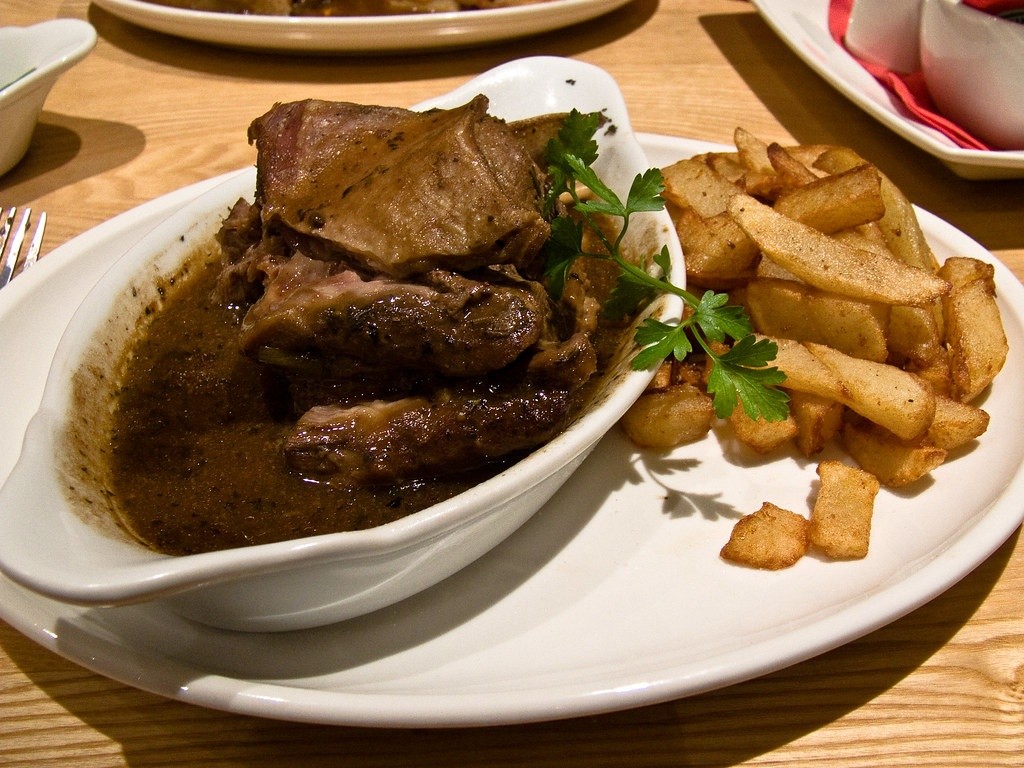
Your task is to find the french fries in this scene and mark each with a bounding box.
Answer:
[622,129,1009,568]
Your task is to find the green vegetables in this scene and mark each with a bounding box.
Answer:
[530,108,791,423]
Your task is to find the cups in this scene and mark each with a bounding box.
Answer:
[844,0,919,73]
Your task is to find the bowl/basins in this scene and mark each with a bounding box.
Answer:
[920,0,1024,151]
[0,56,686,633]
[0,18,97,178]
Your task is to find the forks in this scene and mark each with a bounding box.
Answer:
[0,206,47,290]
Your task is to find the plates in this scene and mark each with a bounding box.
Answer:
[0,130,1024,728]
[749,0,1024,180]
[93,0,633,52]
